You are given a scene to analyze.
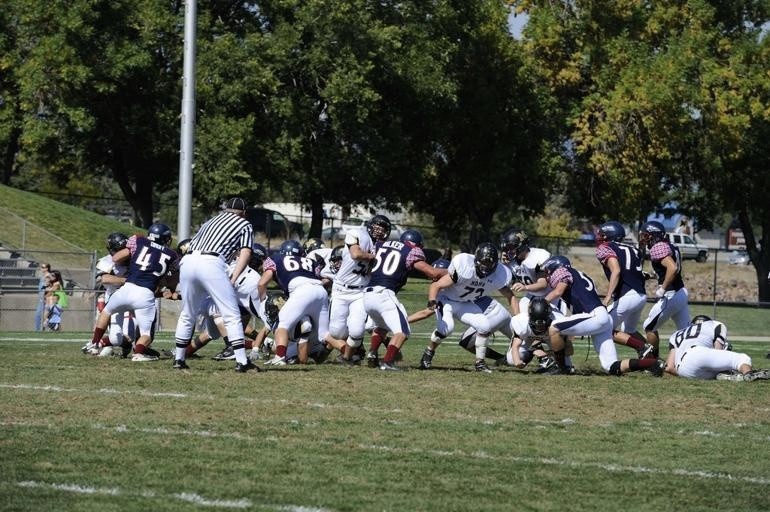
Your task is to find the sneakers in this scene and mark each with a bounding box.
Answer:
[743,369,770,381]
[81,337,194,370]
[420,356,435,372]
[717,373,735,382]
[637,342,655,360]
[535,353,576,375]
[475,359,493,372]
[212,338,400,372]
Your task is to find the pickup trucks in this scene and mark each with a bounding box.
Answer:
[633,232,710,264]
[244,206,305,242]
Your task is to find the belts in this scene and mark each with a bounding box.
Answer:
[677,345,697,372]
[344,284,363,290]
[186,251,220,258]
[364,288,376,291]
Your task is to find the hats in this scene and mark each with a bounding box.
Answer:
[226,197,247,210]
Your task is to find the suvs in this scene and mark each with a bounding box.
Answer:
[336,215,405,245]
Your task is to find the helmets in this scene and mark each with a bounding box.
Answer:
[430,260,450,273]
[544,256,571,275]
[501,230,531,258]
[527,297,552,333]
[252,215,424,274]
[691,314,711,326]
[595,221,626,243]
[106,224,193,258]
[474,242,498,275]
[638,221,665,246]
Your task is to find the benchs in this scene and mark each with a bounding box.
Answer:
[0,251,43,294]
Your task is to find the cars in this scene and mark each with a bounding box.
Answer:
[729,249,752,266]
[303,224,348,241]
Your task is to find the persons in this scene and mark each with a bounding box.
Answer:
[36,263,67,333]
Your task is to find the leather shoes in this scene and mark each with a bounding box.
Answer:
[654,358,668,377]
